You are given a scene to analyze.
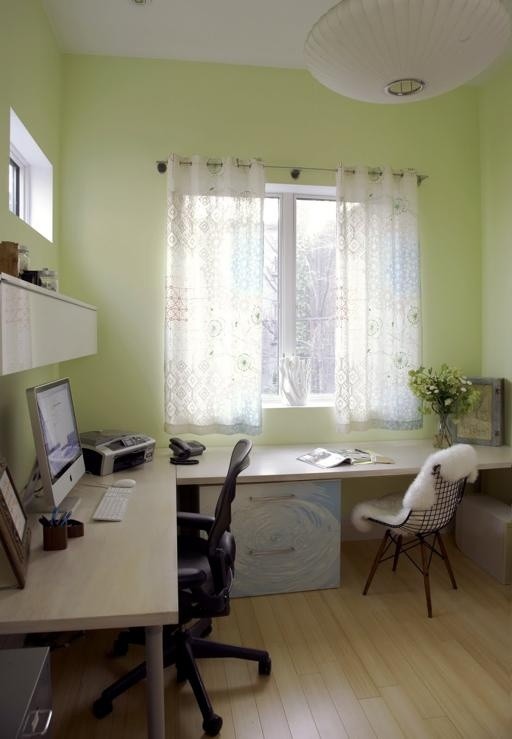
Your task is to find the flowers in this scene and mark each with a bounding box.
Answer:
[408,362,482,444]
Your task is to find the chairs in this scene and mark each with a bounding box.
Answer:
[94,439,270,738]
[351,444,478,619]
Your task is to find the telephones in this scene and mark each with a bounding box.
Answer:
[169,437,206,459]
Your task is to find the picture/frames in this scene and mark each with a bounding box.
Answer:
[448,378,504,447]
[0,463,32,589]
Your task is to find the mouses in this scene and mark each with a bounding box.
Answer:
[112,479,136,488]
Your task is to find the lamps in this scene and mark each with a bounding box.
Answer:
[302,0,511,105]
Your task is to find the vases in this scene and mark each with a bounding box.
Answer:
[432,414,456,449]
[280,356,312,407]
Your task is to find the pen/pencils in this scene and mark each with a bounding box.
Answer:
[52,508,57,527]
[38,515,50,527]
[60,511,72,527]
[58,511,68,525]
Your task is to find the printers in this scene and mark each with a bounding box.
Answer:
[79,430,156,476]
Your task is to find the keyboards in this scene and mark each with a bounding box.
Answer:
[92,486,133,521]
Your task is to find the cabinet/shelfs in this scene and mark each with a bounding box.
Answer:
[1,272,99,381]
[0,647,54,739]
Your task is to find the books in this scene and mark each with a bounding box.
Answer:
[296,446,394,469]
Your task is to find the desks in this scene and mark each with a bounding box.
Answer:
[177,444,512,600]
[0,448,178,736]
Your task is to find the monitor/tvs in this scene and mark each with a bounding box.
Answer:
[26,377,86,512]
